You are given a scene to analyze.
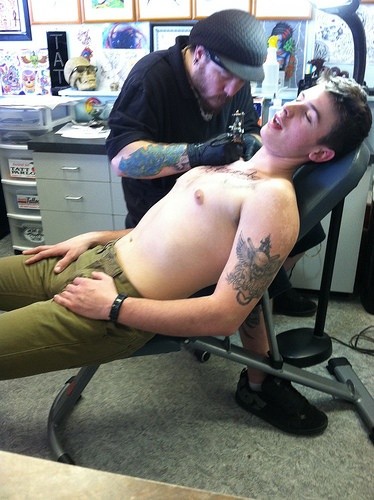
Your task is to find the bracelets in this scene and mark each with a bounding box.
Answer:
[109,294,127,324]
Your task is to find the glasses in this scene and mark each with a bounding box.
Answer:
[68,64,98,86]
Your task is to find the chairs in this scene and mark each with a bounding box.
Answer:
[46,142,374,465]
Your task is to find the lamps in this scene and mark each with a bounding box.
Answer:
[309,0,366,88]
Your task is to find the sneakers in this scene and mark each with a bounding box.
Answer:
[234,367,327,435]
[274,289,316,318]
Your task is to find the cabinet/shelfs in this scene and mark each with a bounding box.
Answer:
[0,105,76,256]
[27,88,374,293]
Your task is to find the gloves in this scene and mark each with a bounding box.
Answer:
[240,133,262,162]
[187,132,240,168]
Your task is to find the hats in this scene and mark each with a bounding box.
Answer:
[189,9,269,82]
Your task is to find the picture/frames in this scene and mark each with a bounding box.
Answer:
[0,0,312,41]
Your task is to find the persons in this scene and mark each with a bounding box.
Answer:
[103,9,328,436]
[0,77,373,381]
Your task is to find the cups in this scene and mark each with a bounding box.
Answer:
[89,103,104,127]
[102,101,114,129]
